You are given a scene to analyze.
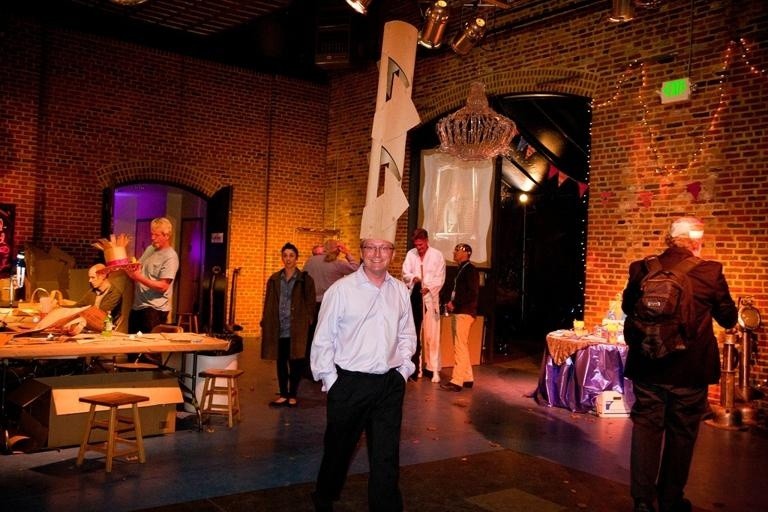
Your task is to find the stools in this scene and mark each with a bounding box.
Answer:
[199,367,243,427]
[178,312,200,333]
[78,392,149,472]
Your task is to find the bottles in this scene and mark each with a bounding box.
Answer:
[104,311,113,338]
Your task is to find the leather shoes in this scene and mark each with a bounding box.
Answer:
[270,398,286,408]
[431,371,442,383]
[463,379,475,388]
[660,499,691,511]
[634,500,655,512]
[288,399,297,406]
[442,382,463,392]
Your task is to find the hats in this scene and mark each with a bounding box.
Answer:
[94,233,140,274]
[671,216,705,247]
[358,170,410,244]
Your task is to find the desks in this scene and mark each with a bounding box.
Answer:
[0,332,230,432]
[0,307,118,332]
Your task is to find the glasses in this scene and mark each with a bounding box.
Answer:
[361,244,396,254]
[457,243,470,253]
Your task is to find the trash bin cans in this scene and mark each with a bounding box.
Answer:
[184,334,243,414]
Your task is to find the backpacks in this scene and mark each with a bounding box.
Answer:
[621,253,705,385]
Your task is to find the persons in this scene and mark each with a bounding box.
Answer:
[622,218,738,511]
[402,228,446,382]
[311,187,417,512]
[124,218,180,369]
[50,261,122,376]
[440,242,480,391]
[304,240,358,331]
[261,244,317,408]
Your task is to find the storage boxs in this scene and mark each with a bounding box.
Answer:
[6,375,184,450]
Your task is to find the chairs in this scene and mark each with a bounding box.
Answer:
[84,313,124,373]
[115,325,183,371]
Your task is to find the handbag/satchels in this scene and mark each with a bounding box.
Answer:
[18,288,52,312]
[593,391,634,419]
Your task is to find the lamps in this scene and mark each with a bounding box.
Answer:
[418,2,449,50]
[436,83,516,161]
